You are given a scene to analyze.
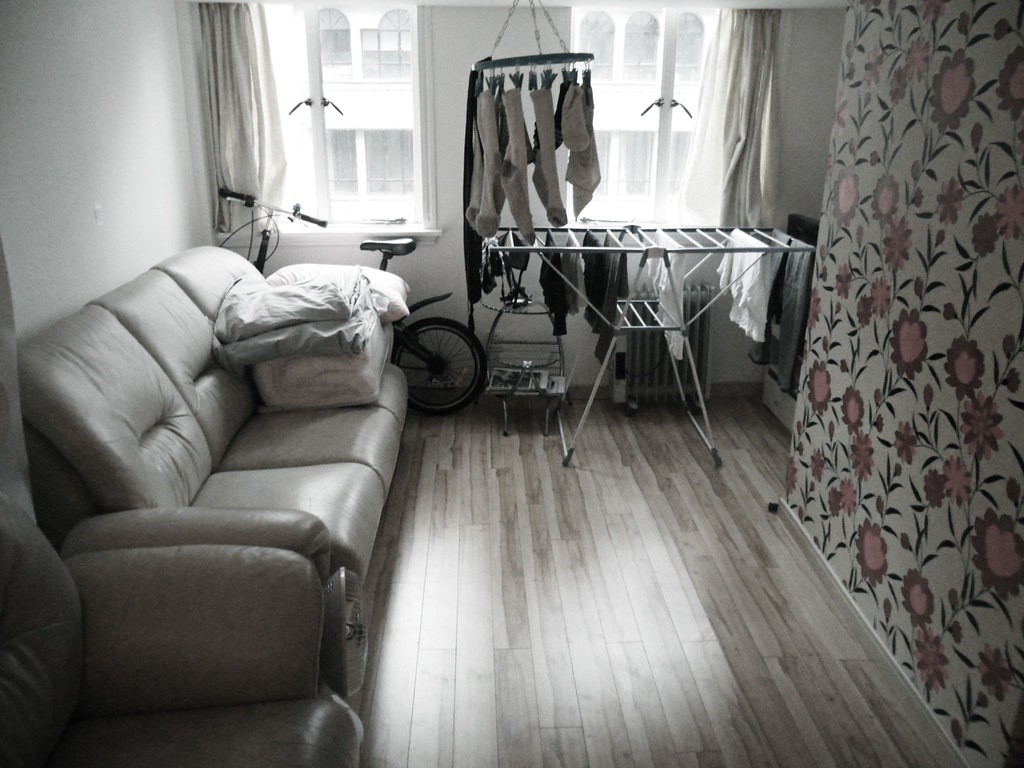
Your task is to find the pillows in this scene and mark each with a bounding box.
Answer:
[264,263,411,323]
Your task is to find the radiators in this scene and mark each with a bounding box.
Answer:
[608,281,714,418]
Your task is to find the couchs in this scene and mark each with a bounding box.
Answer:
[1,492,365,767]
[18,245,424,714]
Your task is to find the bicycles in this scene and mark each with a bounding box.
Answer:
[219,186,487,415]
